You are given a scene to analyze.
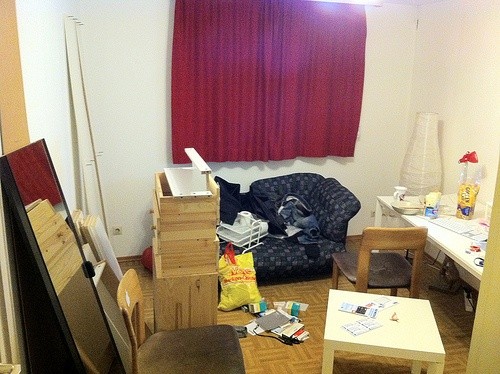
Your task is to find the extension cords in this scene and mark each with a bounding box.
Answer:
[464,290,474,312]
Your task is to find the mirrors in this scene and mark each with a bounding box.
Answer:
[0,137,119,374]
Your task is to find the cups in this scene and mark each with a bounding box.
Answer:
[393,186,408,201]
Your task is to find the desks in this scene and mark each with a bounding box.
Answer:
[321,289,446,374]
[372,194,491,292]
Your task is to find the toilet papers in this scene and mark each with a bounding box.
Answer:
[239,211,251,226]
[258,222,269,234]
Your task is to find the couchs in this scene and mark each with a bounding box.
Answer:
[218,173,361,286]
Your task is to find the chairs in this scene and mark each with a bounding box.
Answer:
[117,269,245,374]
[331,227,428,299]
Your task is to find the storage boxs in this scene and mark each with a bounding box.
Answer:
[249,301,267,313]
[24,197,82,295]
[148,171,220,333]
[284,301,310,317]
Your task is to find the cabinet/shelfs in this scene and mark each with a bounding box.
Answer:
[216,211,268,253]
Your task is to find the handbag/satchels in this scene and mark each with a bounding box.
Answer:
[217,241,262,311]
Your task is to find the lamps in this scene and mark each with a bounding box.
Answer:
[399,113,442,195]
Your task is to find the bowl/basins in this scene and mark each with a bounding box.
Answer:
[392,201,421,214]
